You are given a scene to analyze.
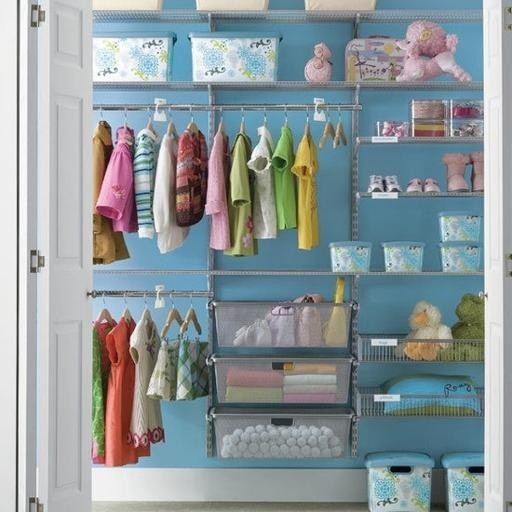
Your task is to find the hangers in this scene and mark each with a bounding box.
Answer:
[87,102,351,151]
[94,290,202,336]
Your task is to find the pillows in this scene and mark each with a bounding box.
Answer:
[377,371,480,415]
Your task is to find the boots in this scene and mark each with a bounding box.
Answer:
[444,152,483,192]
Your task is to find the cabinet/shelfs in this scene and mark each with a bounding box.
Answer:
[75,2,487,511]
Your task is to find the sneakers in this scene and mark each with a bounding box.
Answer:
[407,177,440,192]
[368,175,401,192]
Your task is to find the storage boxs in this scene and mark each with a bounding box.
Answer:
[438,448,484,512]
[205,297,360,353]
[186,30,285,83]
[205,353,362,406]
[362,447,436,512]
[205,407,360,459]
[93,30,178,82]
